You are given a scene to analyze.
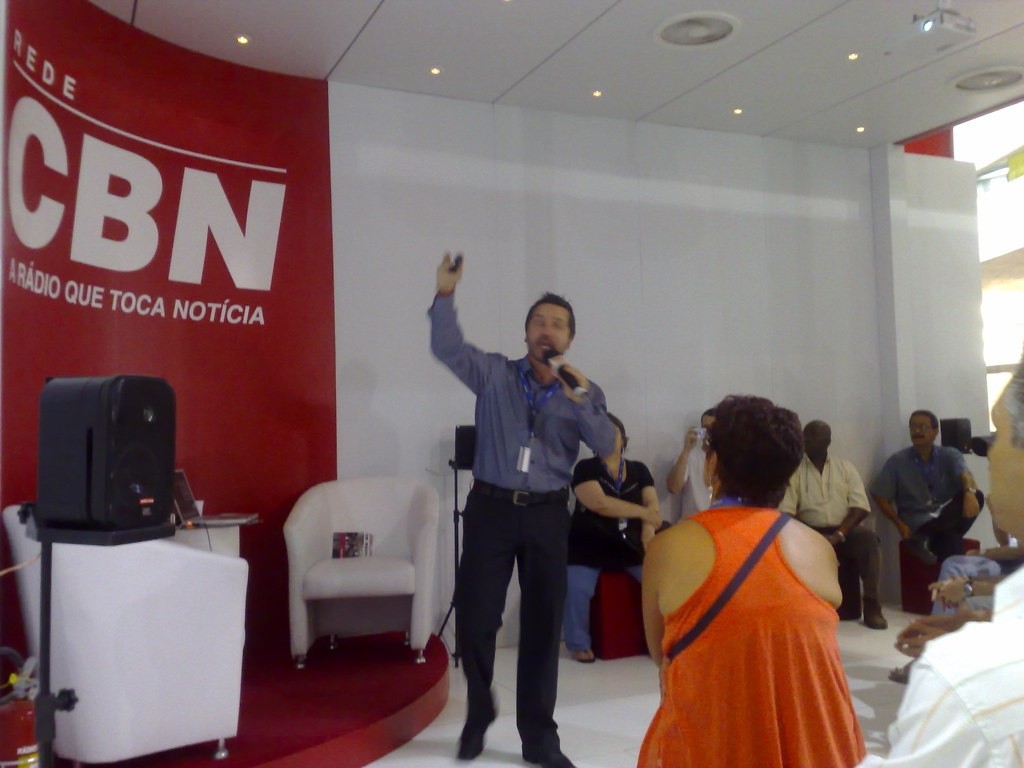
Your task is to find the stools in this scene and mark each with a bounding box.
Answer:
[589,569,646,661]
[895,537,982,615]
[838,568,862,621]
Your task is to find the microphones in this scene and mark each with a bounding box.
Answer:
[544,349,584,396]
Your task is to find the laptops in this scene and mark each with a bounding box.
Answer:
[173,468,259,525]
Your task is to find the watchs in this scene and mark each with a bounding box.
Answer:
[964,578,976,597]
[836,530,846,543]
[963,487,977,494]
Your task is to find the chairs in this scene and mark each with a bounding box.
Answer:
[283,473,443,670]
[2,501,249,768]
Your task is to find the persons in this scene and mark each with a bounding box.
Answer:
[871,410,985,563]
[636,394,868,768]
[889,494,1024,683]
[667,408,718,522]
[562,412,663,664]
[778,420,887,630]
[427,252,618,768]
[851,354,1024,768]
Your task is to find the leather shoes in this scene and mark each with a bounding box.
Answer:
[521,742,576,768]
[457,693,498,759]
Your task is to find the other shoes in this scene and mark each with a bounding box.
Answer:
[907,532,936,563]
[862,612,889,629]
[888,667,908,684]
[571,649,595,663]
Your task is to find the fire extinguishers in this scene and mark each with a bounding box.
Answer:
[0,673,43,768]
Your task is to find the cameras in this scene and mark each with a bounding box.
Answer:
[694,427,707,439]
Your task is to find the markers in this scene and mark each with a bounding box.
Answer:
[450,256,462,272]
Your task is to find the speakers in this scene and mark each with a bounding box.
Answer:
[36,375,175,532]
